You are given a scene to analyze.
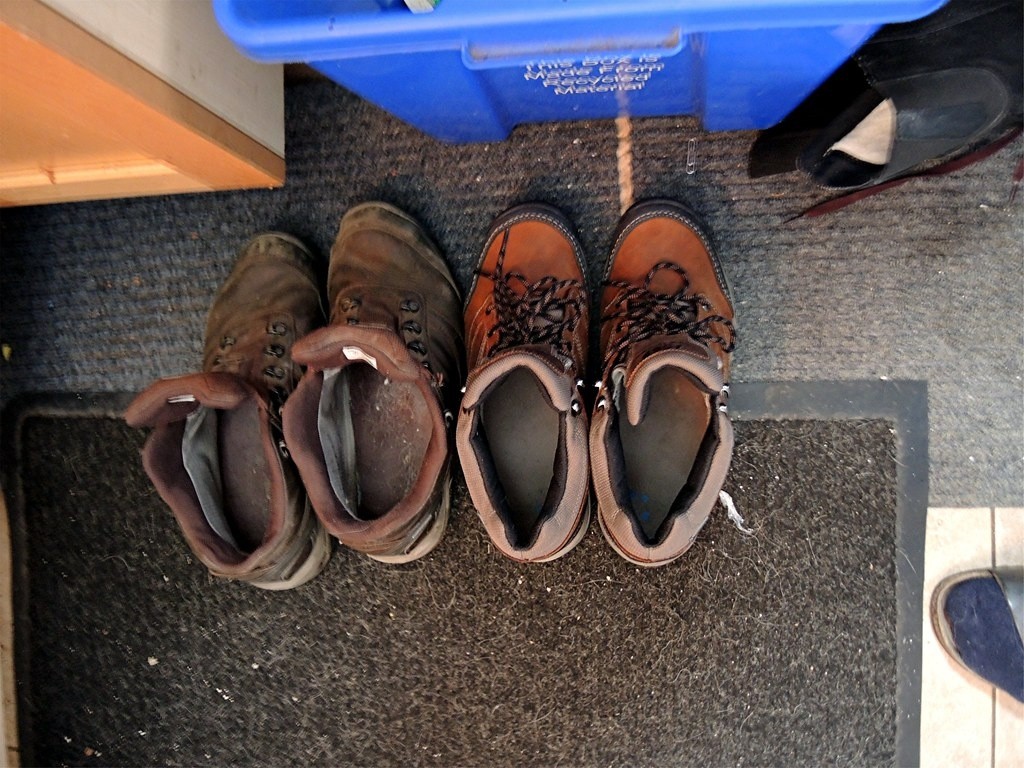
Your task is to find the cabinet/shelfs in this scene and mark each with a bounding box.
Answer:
[0,0,285,209]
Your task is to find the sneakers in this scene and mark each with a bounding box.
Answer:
[281,201,461,563]
[126,233,331,590]
[589,196,737,567]
[456,203,589,563]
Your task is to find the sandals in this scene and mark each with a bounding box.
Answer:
[929,568,1024,707]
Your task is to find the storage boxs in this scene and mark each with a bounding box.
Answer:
[213,0,949,147]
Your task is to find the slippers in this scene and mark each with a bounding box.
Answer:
[795,66,1014,190]
